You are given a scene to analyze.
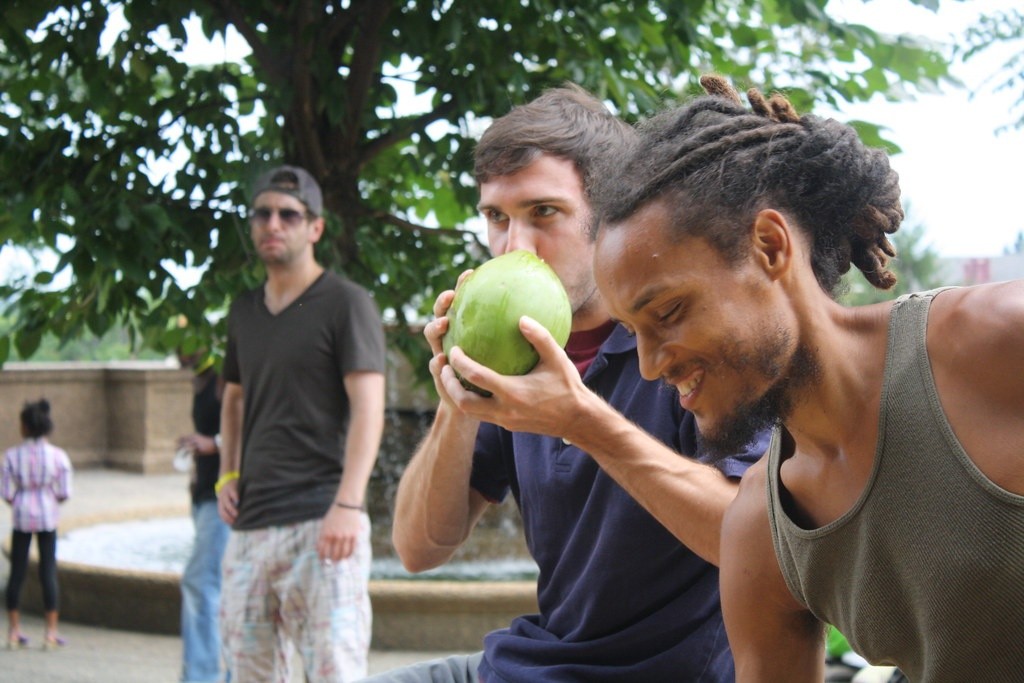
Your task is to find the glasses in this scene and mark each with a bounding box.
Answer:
[250,206,306,224]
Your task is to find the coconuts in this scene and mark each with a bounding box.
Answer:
[441,249,573,398]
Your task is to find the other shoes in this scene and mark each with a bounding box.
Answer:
[43,637,66,651]
[8,634,30,651]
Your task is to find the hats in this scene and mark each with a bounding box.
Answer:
[251,165,323,219]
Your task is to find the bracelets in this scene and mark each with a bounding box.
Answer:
[215,471,241,494]
[214,432,222,450]
[331,498,364,514]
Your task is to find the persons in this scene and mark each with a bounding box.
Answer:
[0,396,72,650]
[212,166,382,681]
[173,311,225,681]
[375,79,772,683]
[591,72,1024,683]
